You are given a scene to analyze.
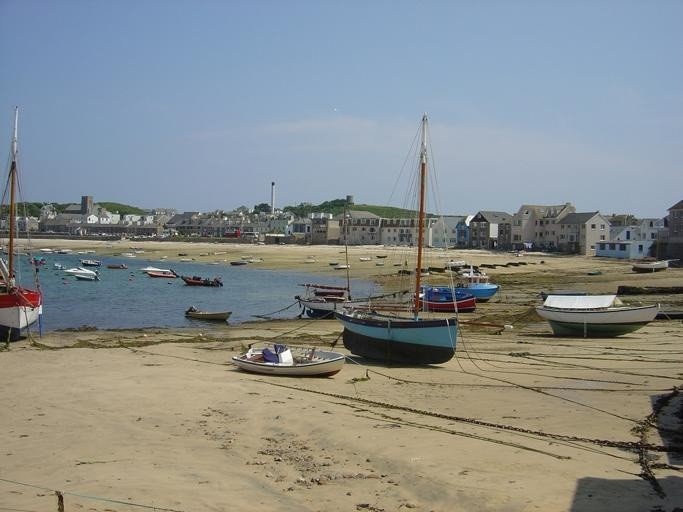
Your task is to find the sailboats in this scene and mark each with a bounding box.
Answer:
[0,103,43,342]
[331,109,460,368]
[627,228,670,274]
[291,202,373,320]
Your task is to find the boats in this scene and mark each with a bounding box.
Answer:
[533,293,661,340]
[410,283,477,313]
[230,340,346,379]
[418,263,502,302]
